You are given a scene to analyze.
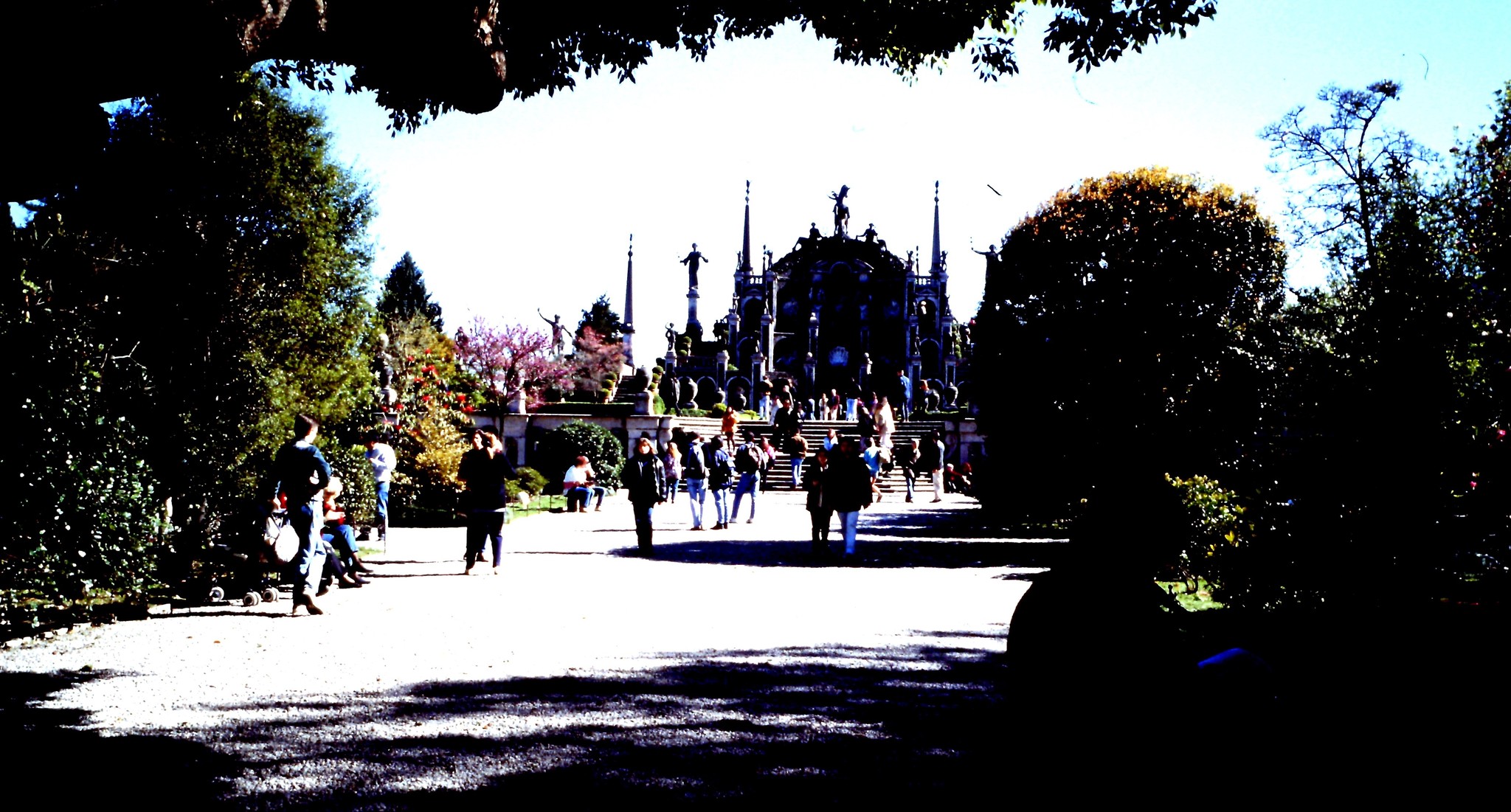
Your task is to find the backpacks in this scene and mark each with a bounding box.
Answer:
[720,456,736,478]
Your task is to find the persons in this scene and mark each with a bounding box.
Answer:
[733,370,936,426]
[732,291,740,314]
[563,454,606,513]
[971,244,1000,295]
[619,438,668,557]
[454,327,469,365]
[249,414,331,615]
[680,431,776,531]
[720,405,739,453]
[766,250,773,270]
[905,251,915,270]
[457,423,513,575]
[680,243,708,290]
[537,307,574,356]
[662,443,684,504]
[735,250,743,271]
[259,475,374,593]
[901,432,972,503]
[791,222,823,250]
[663,377,681,417]
[940,251,948,271]
[802,429,883,564]
[355,429,397,543]
[856,223,878,242]
[826,191,850,219]
[666,323,677,351]
[769,398,808,490]
[856,396,896,478]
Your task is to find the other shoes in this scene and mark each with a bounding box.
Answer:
[348,573,370,584]
[724,522,728,529]
[339,573,361,589]
[690,524,707,530]
[302,588,323,615]
[710,520,723,530]
[729,517,737,523]
[465,569,473,575]
[476,554,489,562]
[905,495,914,503]
[352,562,373,574]
[929,499,947,504]
[463,554,467,560]
[379,535,385,540]
[319,576,335,593]
[355,533,369,541]
[746,518,753,523]
[876,494,883,503]
[292,604,308,616]
[494,567,498,575]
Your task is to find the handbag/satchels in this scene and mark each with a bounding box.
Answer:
[879,449,891,464]
[744,443,762,470]
[821,407,824,411]
[681,469,688,479]
[273,517,299,564]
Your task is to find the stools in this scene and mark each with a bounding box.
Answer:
[567,498,579,512]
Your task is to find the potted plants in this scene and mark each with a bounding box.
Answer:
[648,366,664,392]
[598,371,617,403]
